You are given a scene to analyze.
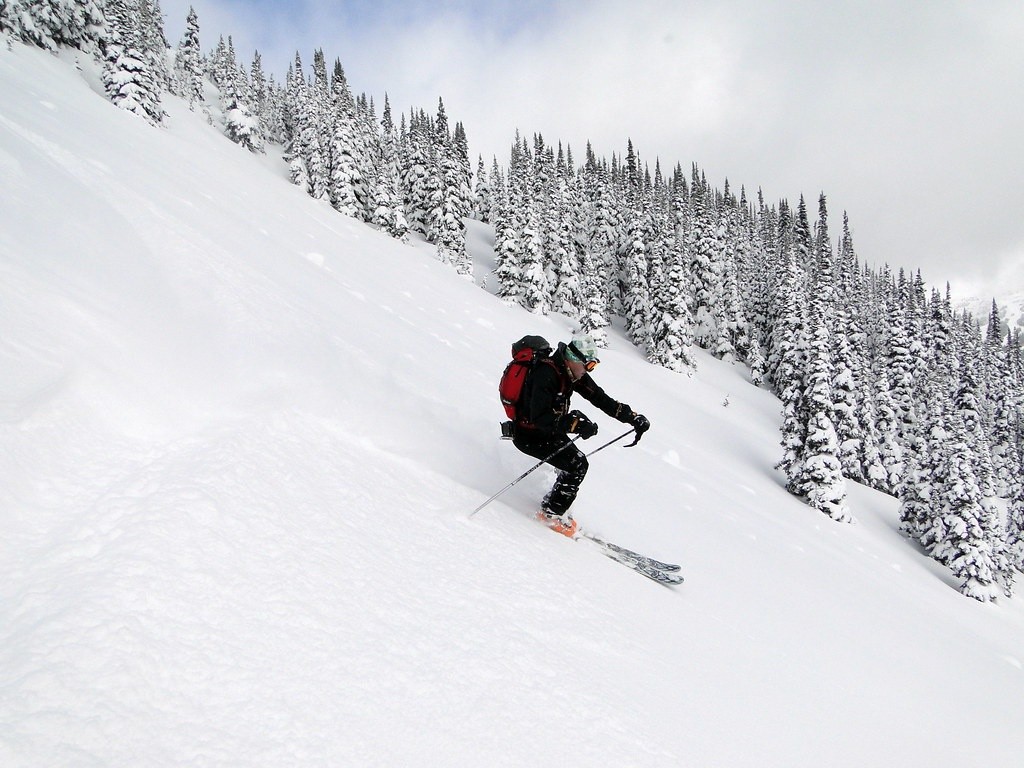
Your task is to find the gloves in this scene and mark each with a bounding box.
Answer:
[573,417,598,440]
[630,412,650,433]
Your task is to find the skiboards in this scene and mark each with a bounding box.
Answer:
[533,506,684,585]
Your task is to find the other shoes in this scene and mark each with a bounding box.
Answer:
[541,500,573,528]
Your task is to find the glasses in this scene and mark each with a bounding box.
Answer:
[583,357,597,372]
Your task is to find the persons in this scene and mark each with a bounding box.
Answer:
[511,335,650,537]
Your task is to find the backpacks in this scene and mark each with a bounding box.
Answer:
[499,335,565,421]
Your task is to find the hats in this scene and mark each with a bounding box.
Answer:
[566,339,598,363]
